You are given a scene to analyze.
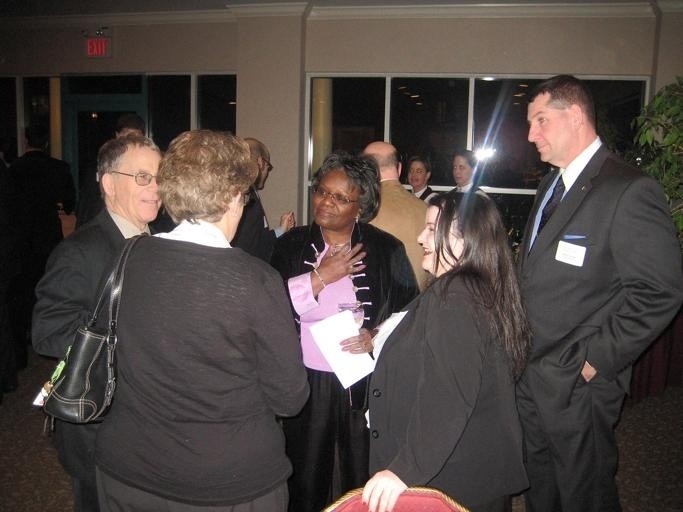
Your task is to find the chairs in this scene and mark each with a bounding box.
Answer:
[57,210,76,238]
[319,487,470,512]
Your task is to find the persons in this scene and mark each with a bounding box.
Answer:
[270,142,534,512]
[515,75,683,512]
[31,117,310,512]
[1,119,77,391]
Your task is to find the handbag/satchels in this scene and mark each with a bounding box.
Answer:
[40,324,118,426]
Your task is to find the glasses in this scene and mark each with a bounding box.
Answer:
[110,169,156,187]
[311,184,359,206]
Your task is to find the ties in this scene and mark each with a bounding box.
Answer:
[528,174,565,252]
